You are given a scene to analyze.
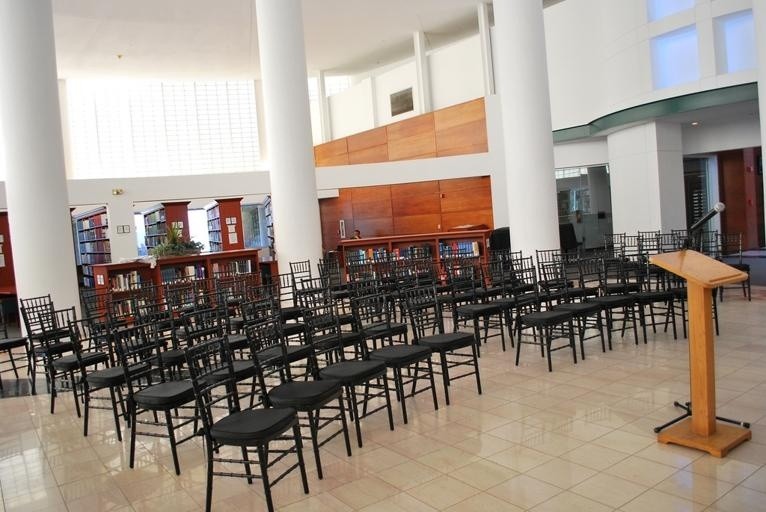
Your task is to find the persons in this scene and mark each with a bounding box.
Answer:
[350,229,362,240]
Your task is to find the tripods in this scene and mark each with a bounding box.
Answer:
[652,398,751,435]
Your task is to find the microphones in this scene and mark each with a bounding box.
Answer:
[689,202,726,231]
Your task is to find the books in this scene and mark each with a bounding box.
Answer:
[77,201,274,320]
[346,239,483,282]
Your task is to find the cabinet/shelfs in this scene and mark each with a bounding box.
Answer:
[338,229,490,289]
[0,196,276,323]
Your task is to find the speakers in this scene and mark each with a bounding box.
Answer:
[489,226,510,252]
